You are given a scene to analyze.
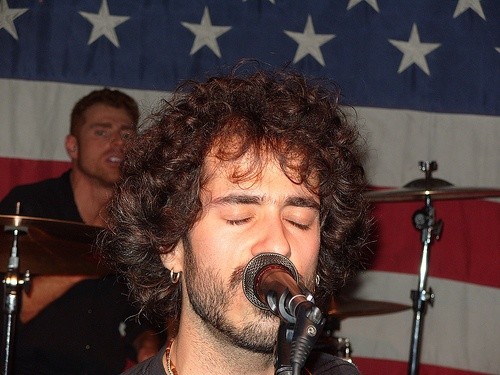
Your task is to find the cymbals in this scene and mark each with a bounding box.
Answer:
[363,186,500,201]
[0,215,111,241]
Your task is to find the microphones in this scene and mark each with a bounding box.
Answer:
[241,253,321,323]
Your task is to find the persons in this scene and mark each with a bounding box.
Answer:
[0,55,381,375]
[0,85,141,346]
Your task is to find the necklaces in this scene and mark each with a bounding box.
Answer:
[166,338,176,375]
[165,336,178,375]
